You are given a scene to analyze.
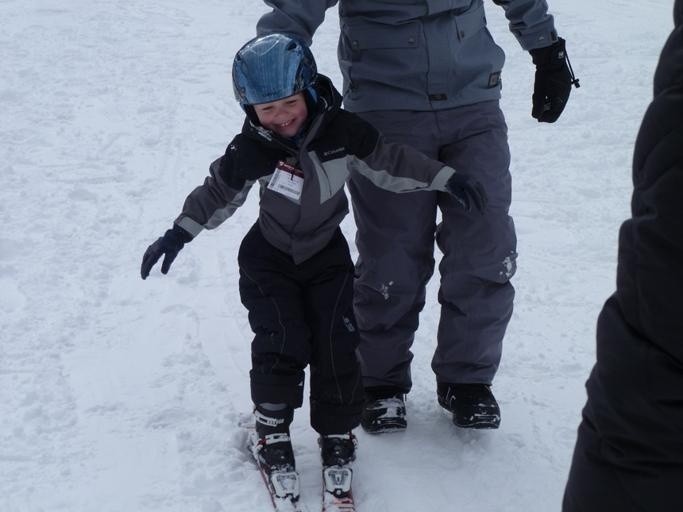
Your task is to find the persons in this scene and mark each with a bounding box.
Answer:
[254,0,575,434]
[139,32,489,502]
[561,1,683,512]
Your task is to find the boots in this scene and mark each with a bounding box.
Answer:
[255,405,295,471]
[319,431,354,466]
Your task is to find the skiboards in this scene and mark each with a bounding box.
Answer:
[248,431,354,512]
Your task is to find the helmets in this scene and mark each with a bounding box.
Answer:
[232,32,317,106]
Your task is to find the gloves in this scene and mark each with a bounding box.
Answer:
[530,37,572,121]
[447,173,488,214]
[141,224,192,279]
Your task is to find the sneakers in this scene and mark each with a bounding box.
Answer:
[437,381,501,429]
[360,392,407,433]
